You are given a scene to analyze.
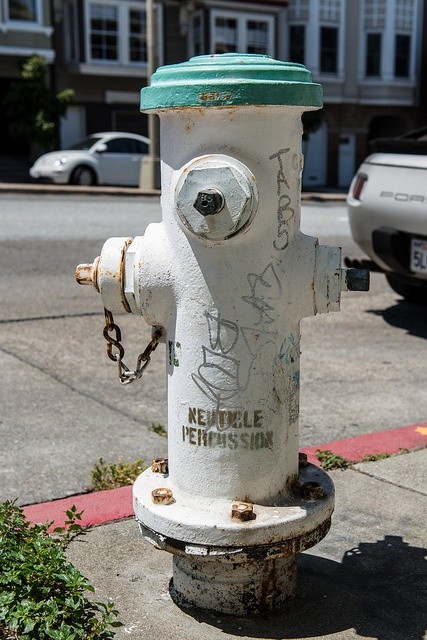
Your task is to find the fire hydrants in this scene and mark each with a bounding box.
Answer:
[75,52,368,617]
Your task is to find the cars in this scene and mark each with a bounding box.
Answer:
[30,132,150,187]
[347,128,427,298]
